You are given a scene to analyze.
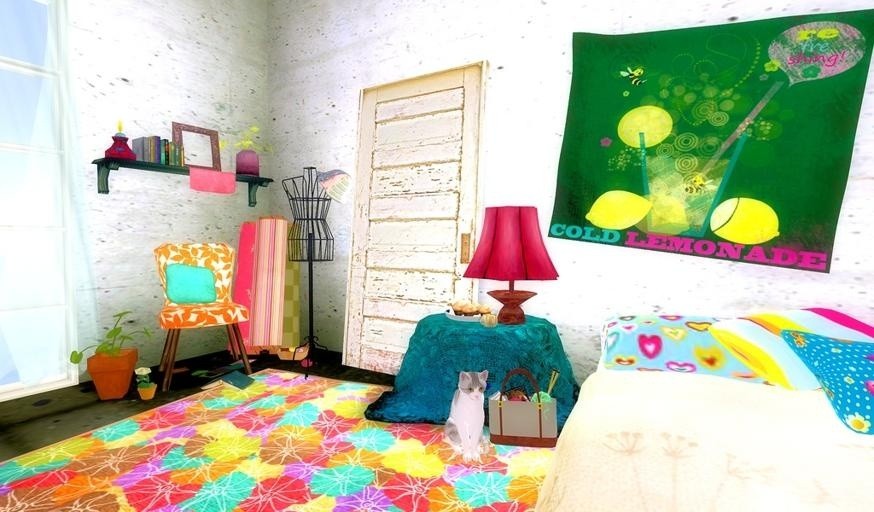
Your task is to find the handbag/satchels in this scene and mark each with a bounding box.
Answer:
[487,395,558,447]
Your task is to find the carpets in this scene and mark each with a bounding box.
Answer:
[0,368,557,512]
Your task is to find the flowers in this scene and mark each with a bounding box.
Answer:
[219,124,273,151]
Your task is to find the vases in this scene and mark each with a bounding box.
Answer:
[236,150,259,177]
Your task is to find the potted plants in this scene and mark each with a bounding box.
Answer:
[70,311,150,401]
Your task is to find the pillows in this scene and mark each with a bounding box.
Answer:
[595,309,873,435]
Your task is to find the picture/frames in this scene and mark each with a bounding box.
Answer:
[172,122,222,171]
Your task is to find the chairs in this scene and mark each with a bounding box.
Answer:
[154,242,255,391]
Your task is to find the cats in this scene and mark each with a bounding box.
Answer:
[444,369,488,463]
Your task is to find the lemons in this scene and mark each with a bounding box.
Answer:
[635,194,688,236]
[711,196,779,246]
[587,189,652,232]
[618,106,672,148]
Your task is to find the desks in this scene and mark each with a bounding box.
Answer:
[367,312,576,431]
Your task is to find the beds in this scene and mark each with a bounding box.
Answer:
[539,306,872,512]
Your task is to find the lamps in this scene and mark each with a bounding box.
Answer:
[466,208,560,325]
